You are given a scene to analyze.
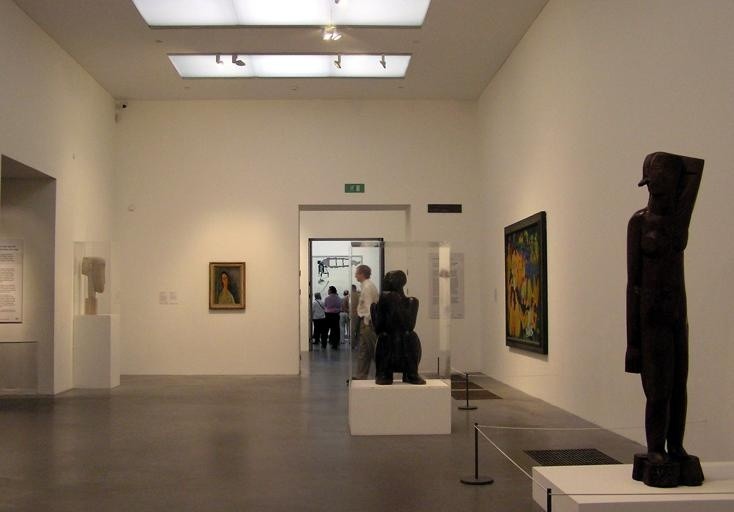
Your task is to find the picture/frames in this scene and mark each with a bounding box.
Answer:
[503,211,548,355]
[208,261,246,310]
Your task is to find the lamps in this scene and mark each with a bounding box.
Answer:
[380,54,387,68]
[216,55,224,64]
[334,54,342,69]
[232,55,246,66]
[320,0,343,41]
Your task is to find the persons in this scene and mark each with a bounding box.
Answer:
[340,290,350,346]
[345,264,378,386]
[313,292,329,350]
[350,284,360,353]
[324,285,342,350]
[216,271,240,304]
